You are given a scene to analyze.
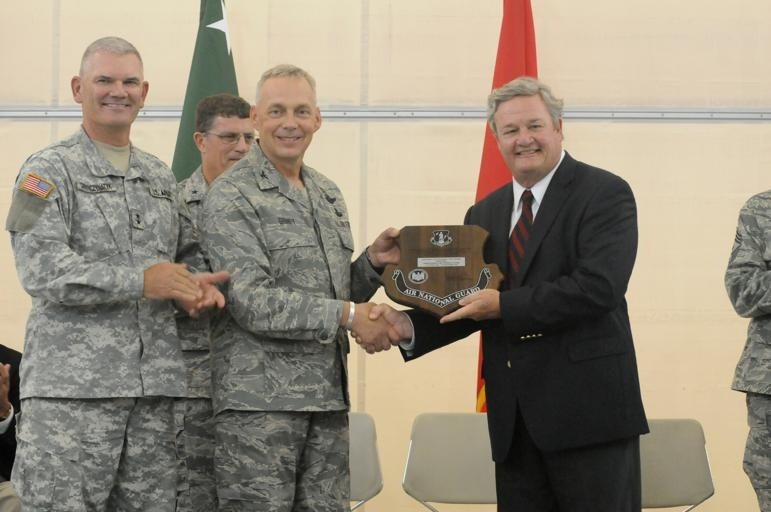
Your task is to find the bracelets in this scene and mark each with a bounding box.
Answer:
[345,301,355,329]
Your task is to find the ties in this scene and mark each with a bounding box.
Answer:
[508,190,533,284]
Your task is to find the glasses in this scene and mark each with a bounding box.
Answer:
[203,130,257,145]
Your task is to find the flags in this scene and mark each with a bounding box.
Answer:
[171,1,239,180]
[474,0,538,414]
[475,331,489,413]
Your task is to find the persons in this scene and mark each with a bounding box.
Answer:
[199,63,401,512]
[0,362,16,481]
[725,189,771,512]
[175,93,255,512]
[6,37,230,512]
[350,76,650,512]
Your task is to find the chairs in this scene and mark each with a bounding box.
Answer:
[348,415,385,512]
[641,418,714,511]
[402,414,497,512]
